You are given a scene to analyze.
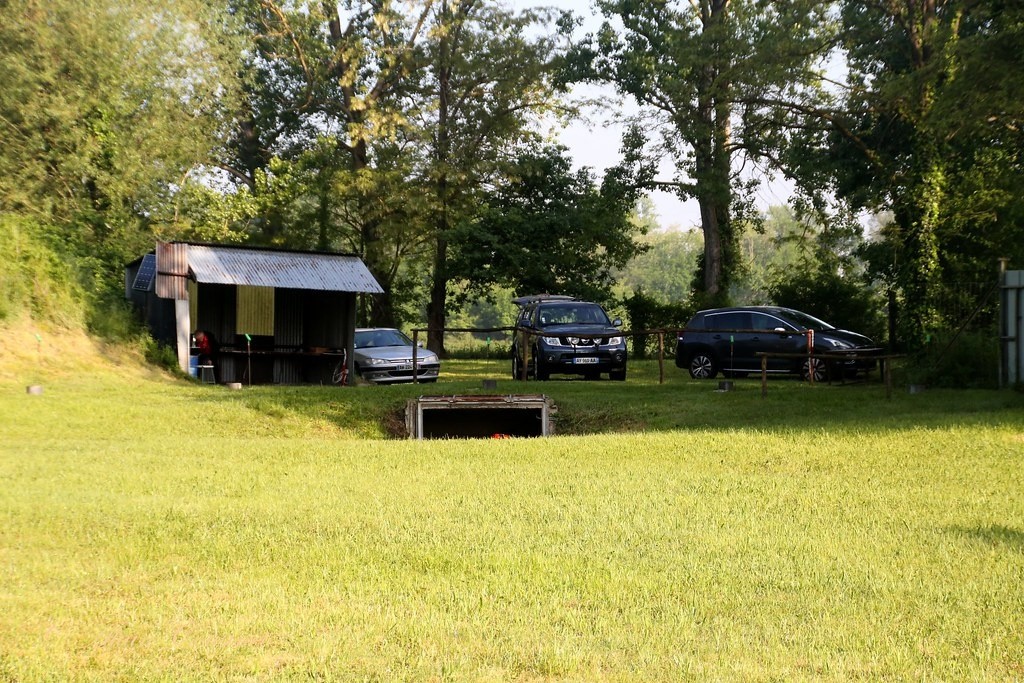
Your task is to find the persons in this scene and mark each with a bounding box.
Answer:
[194,330,222,385]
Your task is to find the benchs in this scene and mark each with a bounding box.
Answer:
[190,365,216,385]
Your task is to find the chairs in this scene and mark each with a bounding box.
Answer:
[541,313,556,323]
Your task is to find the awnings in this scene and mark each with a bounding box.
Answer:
[184,245,385,294]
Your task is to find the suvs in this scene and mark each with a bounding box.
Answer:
[511,294,628,381]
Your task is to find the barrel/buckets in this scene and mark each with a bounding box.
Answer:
[190,355,198,378]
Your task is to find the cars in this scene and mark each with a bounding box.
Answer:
[674,305,878,384]
[354,327,440,385]
